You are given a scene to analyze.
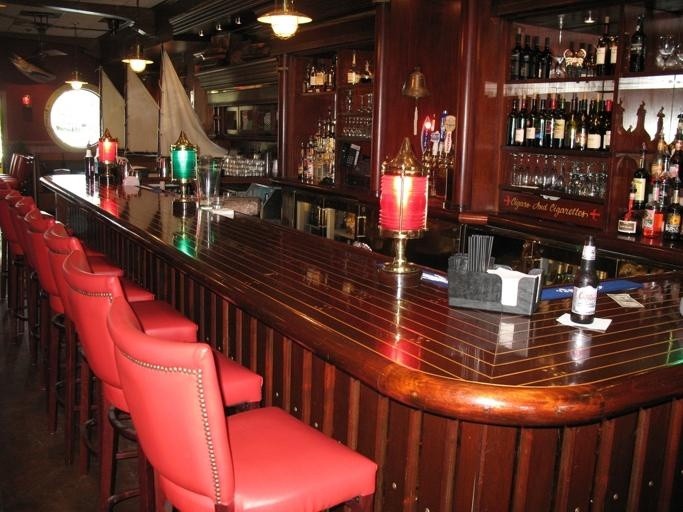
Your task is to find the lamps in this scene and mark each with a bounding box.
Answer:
[376,276,422,370]
[257,0,313,39]
[22,94,33,122]
[65,25,89,90]
[121,0,155,73]
[170,130,200,209]
[98,127,119,184]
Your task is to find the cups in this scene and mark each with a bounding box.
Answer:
[195,153,225,210]
[159,158,170,179]
[222,153,269,177]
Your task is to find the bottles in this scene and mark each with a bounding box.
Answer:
[501,8,683,247]
[301,49,373,93]
[340,88,374,141]
[295,101,336,188]
[83,149,95,178]
[423,106,461,202]
[85,175,95,197]
[565,327,593,386]
[543,259,608,289]
[569,230,598,324]
